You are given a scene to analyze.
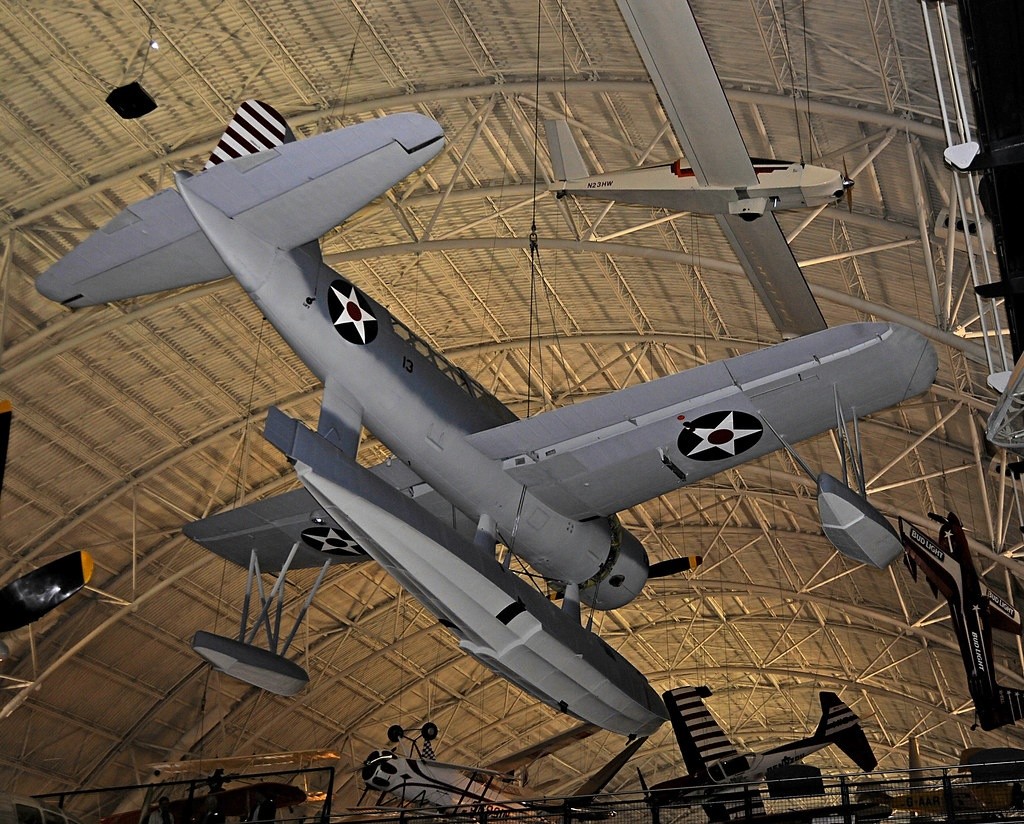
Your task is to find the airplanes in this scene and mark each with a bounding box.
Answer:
[0,1,1023,823]
